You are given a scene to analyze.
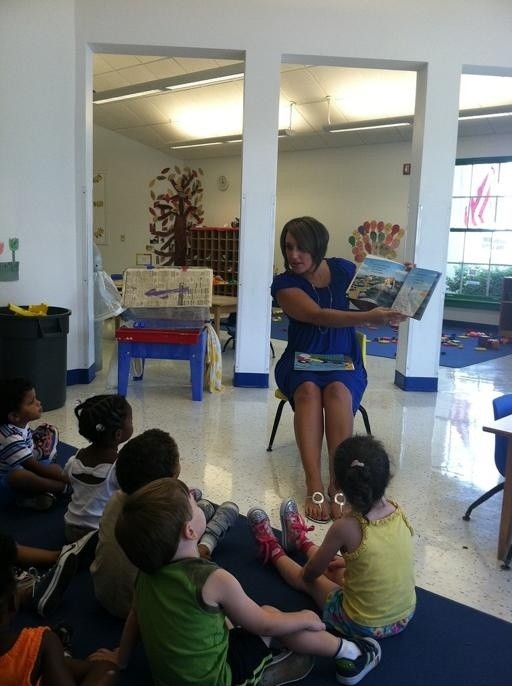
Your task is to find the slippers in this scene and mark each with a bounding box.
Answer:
[329,485,352,522]
[304,489,332,525]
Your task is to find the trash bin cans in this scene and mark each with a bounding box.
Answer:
[0,304,73,413]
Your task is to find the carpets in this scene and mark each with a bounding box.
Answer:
[0,430,512,686]
[273,311,512,371]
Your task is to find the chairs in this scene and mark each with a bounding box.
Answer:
[463,393,512,519]
[266,329,372,452]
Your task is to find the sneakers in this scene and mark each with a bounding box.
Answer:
[57,529,98,571]
[196,498,215,523]
[44,488,57,505]
[246,507,281,565]
[205,500,239,545]
[38,425,59,464]
[188,486,202,500]
[63,483,73,503]
[280,497,306,556]
[34,553,78,618]
[31,422,48,444]
[54,625,74,659]
[334,635,382,686]
[260,649,316,686]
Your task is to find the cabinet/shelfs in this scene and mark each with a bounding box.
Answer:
[188,226,242,297]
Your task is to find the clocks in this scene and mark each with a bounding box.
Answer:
[216,174,229,191]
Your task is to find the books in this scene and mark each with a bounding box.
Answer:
[293,351,355,371]
[346,253,442,321]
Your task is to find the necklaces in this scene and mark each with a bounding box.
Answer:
[311,282,333,335]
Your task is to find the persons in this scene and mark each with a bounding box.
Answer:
[270,216,417,525]
[90,428,239,621]
[0,379,74,510]
[15,529,99,618]
[247,435,417,640]
[85,477,381,686]
[0,534,120,686]
[62,393,203,544]
